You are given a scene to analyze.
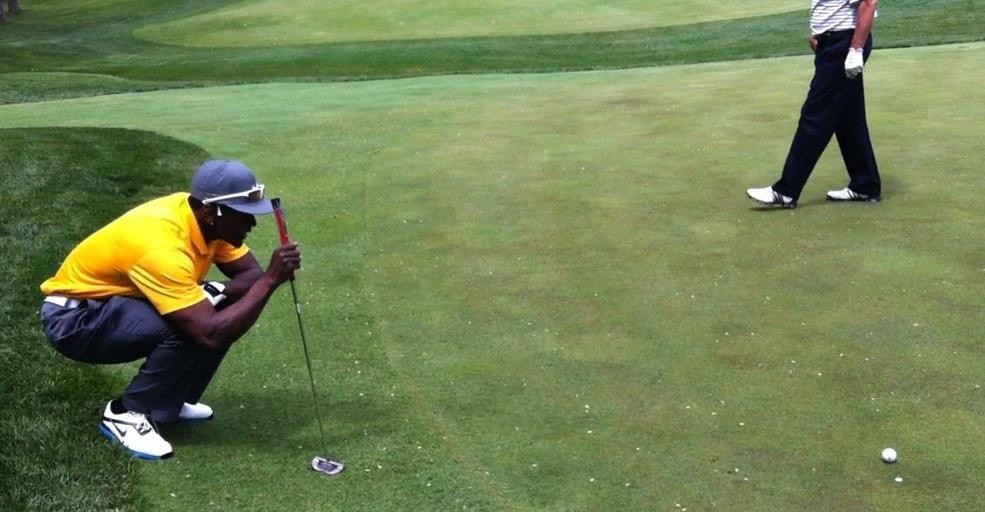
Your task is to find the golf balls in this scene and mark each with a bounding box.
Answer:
[880,448,897,462]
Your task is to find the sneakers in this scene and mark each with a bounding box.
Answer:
[98,399,173,459]
[178,401,213,420]
[746,186,797,208]
[826,186,880,203]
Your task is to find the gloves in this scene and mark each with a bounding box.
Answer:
[199,280,228,308]
[844,47,864,80]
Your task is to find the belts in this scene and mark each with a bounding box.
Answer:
[44,295,82,308]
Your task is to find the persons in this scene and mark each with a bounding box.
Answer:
[747,0,882,208]
[39,161,301,460]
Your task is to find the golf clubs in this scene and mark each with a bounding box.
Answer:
[270,197,343,475]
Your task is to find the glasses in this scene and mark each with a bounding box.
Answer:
[201,184,265,208]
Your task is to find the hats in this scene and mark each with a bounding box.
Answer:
[190,159,275,215]
